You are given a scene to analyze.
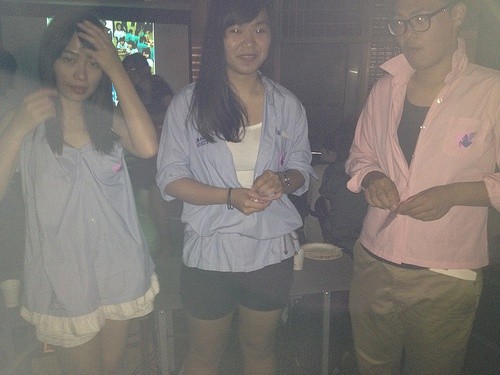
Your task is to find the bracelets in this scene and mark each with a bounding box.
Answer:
[227,188,233,210]
[279,171,290,195]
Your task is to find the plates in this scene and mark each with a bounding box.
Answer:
[301,243,343,261]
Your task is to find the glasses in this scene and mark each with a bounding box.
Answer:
[386,2,452,37]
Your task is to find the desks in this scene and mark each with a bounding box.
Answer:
[289,253,354,375]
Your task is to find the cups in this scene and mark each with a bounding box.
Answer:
[294,249,304,271]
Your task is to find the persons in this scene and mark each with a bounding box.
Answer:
[347,0,500,375]
[0,6,175,375]
[155,0,313,375]
